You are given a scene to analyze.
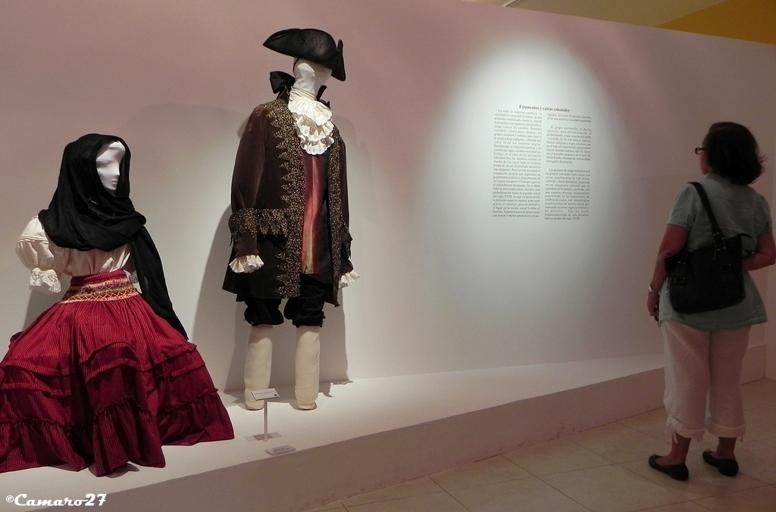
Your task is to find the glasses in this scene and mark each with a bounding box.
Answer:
[695,146,706,155]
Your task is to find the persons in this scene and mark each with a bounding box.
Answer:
[220,23,355,414]
[0,130,235,479]
[642,119,774,485]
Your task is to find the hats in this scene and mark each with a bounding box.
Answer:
[263,28,346,82]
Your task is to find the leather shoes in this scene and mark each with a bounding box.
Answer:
[703,450,739,477]
[648,453,690,480]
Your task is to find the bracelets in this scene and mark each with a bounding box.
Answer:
[648,281,656,294]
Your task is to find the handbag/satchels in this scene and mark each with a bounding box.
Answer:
[664,180,752,314]
